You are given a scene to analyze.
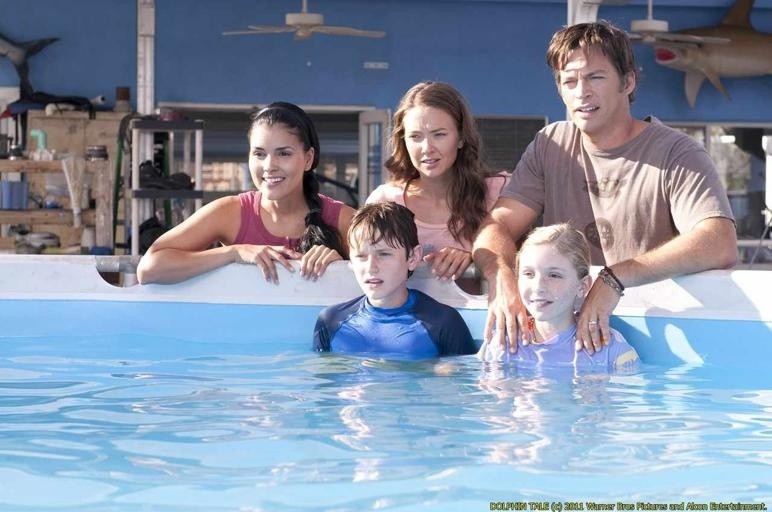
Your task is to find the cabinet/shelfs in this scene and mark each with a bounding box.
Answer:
[0,109,205,255]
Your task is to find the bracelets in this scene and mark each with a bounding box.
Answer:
[596,265,626,297]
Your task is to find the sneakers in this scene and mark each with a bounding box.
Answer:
[139,162,195,190]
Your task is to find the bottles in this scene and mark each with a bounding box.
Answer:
[6,144,24,183]
[83,144,109,209]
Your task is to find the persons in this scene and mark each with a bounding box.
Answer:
[311,199,478,358]
[134,101,360,286]
[475,220,642,370]
[467,17,743,364]
[365,76,516,285]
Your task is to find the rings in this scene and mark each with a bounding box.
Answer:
[588,319,598,325]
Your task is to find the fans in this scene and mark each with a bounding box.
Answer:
[222,0,385,39]
[625,0,731,49]
